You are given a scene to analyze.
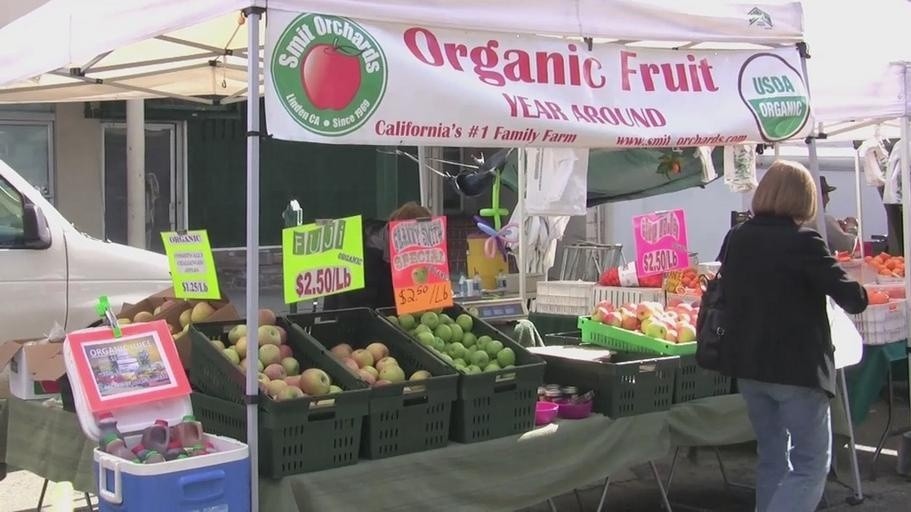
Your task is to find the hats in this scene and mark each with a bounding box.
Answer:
[820,176,836,194]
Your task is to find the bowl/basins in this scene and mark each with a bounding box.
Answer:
[558,397,594,420]
[534,402,559,425]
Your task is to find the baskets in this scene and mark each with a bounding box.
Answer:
[376,302,549,443]
[673,353,731,404]
[544,331,581,346]
[578,315,697,358]
[526,344,680,421]
[190,389,363,475]
[190,317,371,415]
[285,306,461,459]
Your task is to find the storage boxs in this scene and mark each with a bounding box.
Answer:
[63,320,252,512]
[0,336,63,400]
[153,293,240,370]
[100,287,181,326]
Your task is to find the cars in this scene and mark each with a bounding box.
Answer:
[0,157,183,349]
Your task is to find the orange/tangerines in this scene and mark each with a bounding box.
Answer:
[866,249,904,280]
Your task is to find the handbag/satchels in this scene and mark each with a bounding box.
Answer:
[696,270,740,374]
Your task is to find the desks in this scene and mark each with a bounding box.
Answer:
[2,340,909,512]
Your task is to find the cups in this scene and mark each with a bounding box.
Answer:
[463,279,473,296]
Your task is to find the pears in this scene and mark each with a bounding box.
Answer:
[100,296,219,344]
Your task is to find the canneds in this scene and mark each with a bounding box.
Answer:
[561,384,578,405]
[543,389,562,404]
[545,382,561,391]
[536,387,546,402]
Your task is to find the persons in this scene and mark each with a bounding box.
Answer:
[802,176,859,256]
[321,200,433,322]
[716,161,870,512]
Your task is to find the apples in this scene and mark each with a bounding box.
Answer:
[328,342,432,394]
[411,267,431,286]
[385,305,515,375]
[300,37,369,112]
[678,265,714,300]
[257,308,343,402]
[208,323,247,377]
[591,299,701,343]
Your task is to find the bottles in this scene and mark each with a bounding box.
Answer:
[473,272,481,296]
[459,272,466,296]
[495,269,506,297]
